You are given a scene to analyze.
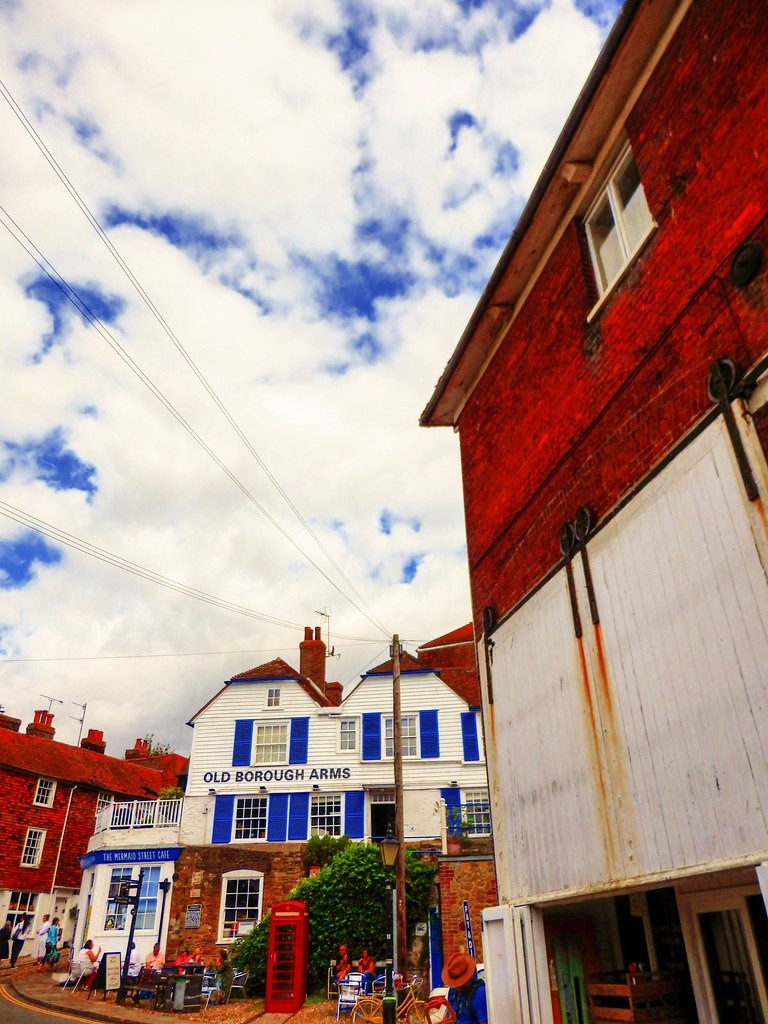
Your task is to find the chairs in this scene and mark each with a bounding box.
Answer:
[159,962,249,1010]
[337,972,386,1021]
[62,960,99,993]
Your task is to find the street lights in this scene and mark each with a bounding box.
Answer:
[377,823,398,1024]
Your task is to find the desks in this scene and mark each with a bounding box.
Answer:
[97,960,130,964]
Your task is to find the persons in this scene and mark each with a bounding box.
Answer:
[35,914,62,972]
[146,942,166,973]
[77,938,101,991]
[212,949,235,1000]
[441,952,489,1024]
[332,943,353,989]
[173,946,193,972]
[358,947,375,995]
[121,942,141,977]
[0,912,34,969]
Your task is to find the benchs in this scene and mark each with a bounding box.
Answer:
[328,958,394,999]
[116,966,174,1010]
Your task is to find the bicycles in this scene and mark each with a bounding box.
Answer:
[350,975,425,1024]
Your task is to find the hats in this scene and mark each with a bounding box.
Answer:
[441,952,476,988]
[180,946,188,952]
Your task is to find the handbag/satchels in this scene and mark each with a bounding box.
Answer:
[46,947,61,964]
[12,923,20,940]
[393,974,403,991]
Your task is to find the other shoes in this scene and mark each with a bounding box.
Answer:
[84,986,90,991]
[11,964,18,969]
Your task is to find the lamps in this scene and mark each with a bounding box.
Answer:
[313,785,320,789]
[450,781,459,785]
[209,788,215,792]
[260,786,266,789]
